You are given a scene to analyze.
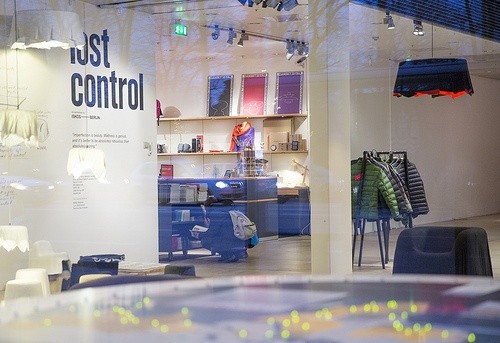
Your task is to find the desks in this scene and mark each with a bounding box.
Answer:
[0,273,500,343]
[118,260,167,275]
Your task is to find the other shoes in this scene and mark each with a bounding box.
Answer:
[238,253,248,260]
[218,254,237,262]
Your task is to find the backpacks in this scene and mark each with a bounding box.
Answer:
[215,207,256,240]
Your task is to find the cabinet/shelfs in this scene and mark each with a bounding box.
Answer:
[158,176,310,262]
[157,113,309,156]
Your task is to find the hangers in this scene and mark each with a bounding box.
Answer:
[358,151,404,168]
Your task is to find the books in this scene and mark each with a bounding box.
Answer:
[173,208,207,251]
[158,181,209,203]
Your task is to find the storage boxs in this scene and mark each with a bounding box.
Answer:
[263,117,307,151]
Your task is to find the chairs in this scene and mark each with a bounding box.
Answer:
[393,226,494,278]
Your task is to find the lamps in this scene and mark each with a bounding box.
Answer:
[238,0,299,11]
[67,0,104,182]
[285,39,309,61]
[9,0,86,50]
[0,148,29,253]
[382,11,395,29]
[211,29,221,40]
[237,33,248,47]
[227,32,236,45]
[0,0,39,148]
[413,19,424,36]
[391,24,474,100]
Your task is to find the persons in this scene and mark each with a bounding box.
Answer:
[190,193,259,263]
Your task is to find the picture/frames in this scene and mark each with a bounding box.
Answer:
[240,73,268,116]
[274,71,304,115]
[207,75,234,117]
